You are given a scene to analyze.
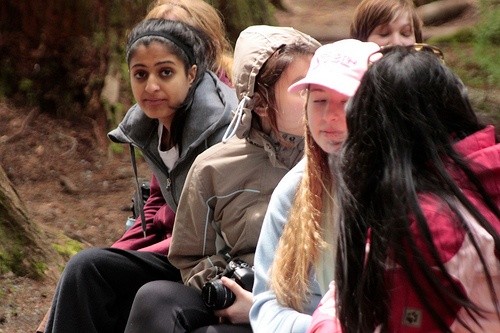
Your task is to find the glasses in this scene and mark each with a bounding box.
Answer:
[366,42,445,67]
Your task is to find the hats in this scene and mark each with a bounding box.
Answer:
[286,37,382,95]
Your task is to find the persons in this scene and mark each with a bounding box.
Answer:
[306,43,500,332]
[36,0,231,333]
[120,24,323,333]
[212,39,384,332]
[350,0,424,47]
[45,17,240,331]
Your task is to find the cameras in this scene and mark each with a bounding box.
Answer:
[202,259,254,309]
